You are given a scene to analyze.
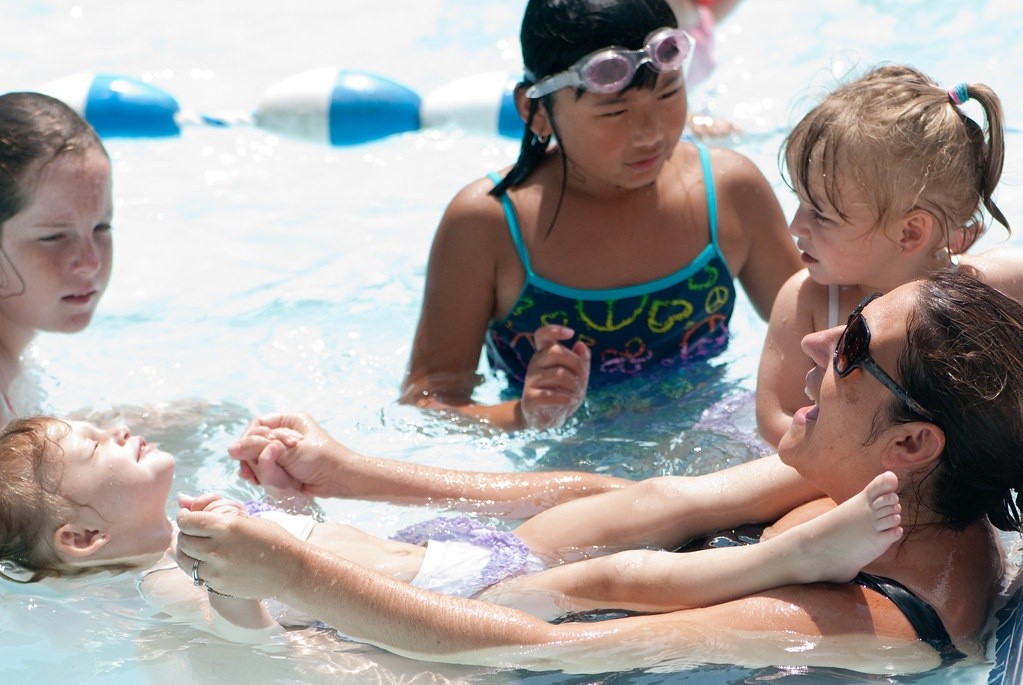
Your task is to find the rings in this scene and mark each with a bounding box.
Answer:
[190,559,206,589]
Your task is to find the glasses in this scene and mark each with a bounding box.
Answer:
[525,28,695,89]
[836,292,935,419]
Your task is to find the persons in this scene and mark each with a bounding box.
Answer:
[401,0,815,444]
[754,61,1023,462]
[1,411,905,661]
[174,269,1019,682]
[0,90,125,409]
